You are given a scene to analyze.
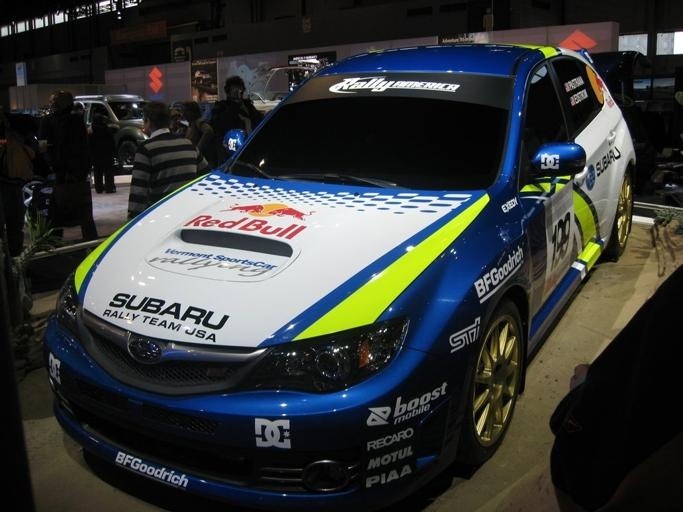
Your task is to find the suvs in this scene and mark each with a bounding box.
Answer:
[193,69,212,85]
[70,92,156,173]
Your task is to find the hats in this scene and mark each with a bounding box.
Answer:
[224,75,245,91]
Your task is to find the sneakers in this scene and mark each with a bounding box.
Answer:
[106,189,116,193]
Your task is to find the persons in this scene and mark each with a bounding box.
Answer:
[0,87,215,259]
[211,73,264,170]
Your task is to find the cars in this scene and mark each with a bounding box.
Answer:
[547,261,682,511]
[42,38,639,512]
